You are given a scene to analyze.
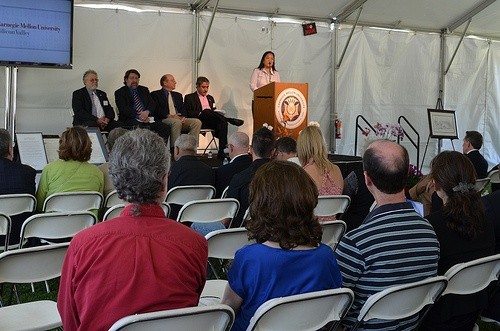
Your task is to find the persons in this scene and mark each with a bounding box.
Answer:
[115,70,161,135]
[184,77,244,162]
[166,126,376,241]
[335,139,441,331]
[37,127,104,245]
[98,128,130,198]
[221,161,342,331]
[419,151,500,331]
[72,70,119,139]
[407,131,488,216]
[0,129,36,252]
[249,51,280,118]
[150,74,202,157]
[57,129,208,331]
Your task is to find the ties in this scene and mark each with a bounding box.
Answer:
[168,91,176,115]
[93,91,106,118]
[133,91,143,112]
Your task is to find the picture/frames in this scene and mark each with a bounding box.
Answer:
[427,109,458,139]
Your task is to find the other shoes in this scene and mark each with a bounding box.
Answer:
[230,117,244,126]
[217,151,231,162]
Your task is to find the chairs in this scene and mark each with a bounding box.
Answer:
[0,185,500,331]
[200,129,218,154]
